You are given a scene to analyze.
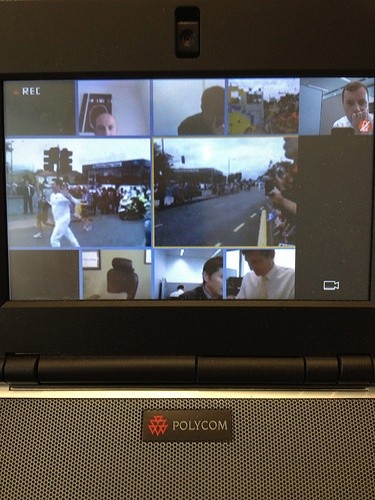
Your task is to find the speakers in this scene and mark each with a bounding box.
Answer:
[0,390,373,500]
[82,93,113,132]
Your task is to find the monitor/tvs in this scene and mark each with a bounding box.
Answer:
[0,70,374,388]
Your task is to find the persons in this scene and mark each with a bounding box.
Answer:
[333,81,373,135]
[8,86,299,298]
